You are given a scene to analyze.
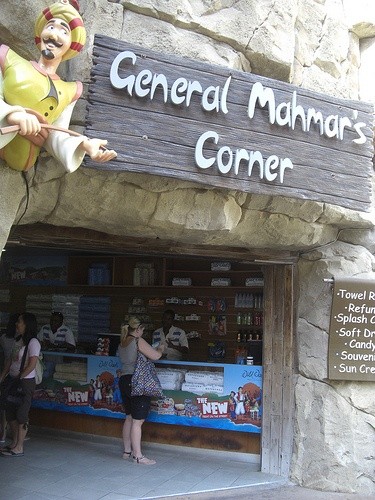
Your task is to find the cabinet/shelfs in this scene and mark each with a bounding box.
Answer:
[0,249,263,366]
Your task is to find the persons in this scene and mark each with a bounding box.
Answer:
[0,0,117,174]
[151,309,189,360]
[117,316,162,465]
[36,311,76,354]
[0,312,41,456]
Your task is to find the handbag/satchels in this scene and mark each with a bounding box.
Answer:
[0,375,24,407]
[130,337,163,398]
[34,352,43,384]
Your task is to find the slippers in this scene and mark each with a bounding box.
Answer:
[0,446,12,452]
[0,451,24,457]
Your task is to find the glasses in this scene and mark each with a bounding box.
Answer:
[137,322,142,328]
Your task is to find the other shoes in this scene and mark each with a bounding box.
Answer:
[24,437,31,440]
[0,440,6,442]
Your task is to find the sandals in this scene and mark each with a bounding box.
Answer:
[132,456,157,465]
[123,451,132,458]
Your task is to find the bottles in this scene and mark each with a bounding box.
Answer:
[238,328,260,342]
[237,311,263,325]
[247,356,253,365]
